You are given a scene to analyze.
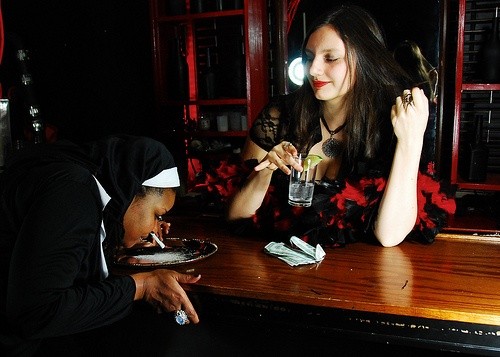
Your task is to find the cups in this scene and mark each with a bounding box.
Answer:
[200,110,248,132]
[287,153,317,207]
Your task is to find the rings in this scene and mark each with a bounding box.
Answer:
[283,142,293,148]
[174,310,188,325]
[402,93,414,104]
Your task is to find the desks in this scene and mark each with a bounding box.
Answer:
[110,224,500,357]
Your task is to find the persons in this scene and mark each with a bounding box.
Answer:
[187,7,458,247]
[0,135,202,357]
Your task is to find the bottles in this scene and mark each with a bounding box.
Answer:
[216,142,235,165]
[466,114,488,182]
[202,47,219,99]
[483,7,500,83]
[238,42,249,91]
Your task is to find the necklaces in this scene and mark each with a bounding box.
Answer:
[319,112,346,158]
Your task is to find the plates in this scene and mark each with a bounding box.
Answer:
[114,237,218,266]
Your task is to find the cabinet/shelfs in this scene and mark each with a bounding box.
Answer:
[151,0,270,140]
[450,0,500,194]
